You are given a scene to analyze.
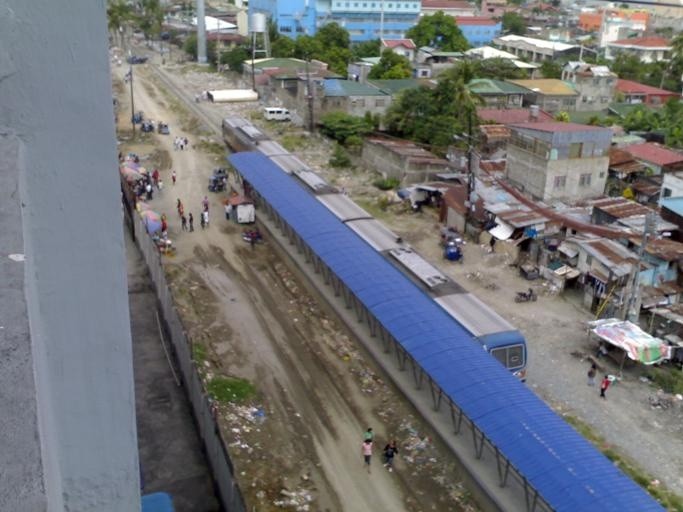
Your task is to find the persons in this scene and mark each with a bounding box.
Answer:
[360,439,373,474]
[134,168,210,241]
[202,89,208,99]
[365,428,375,442]
[173,136,188,151]
[600,375,609,398]
[224,202,231,220]
[221,176,227,191]
[383,440,399,472]
[251,232,256,251]
[596,342,608,358]
[586,363,596,385]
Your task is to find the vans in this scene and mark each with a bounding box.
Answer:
[264,107,292,122]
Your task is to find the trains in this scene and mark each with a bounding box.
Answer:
[221,114,528,384]
